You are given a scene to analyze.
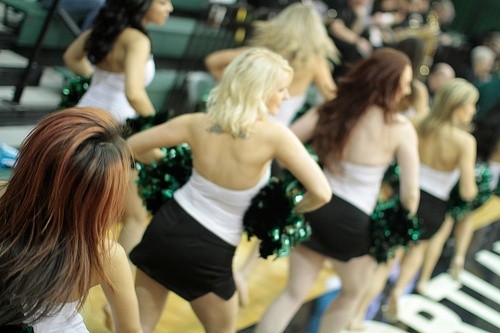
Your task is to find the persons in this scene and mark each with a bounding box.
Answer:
[201,2,337,307]
[0,105,142,333]
[253,48,421,333]
[126,47,332,333]
[63,0,174,333]
[303,0,500,333]
